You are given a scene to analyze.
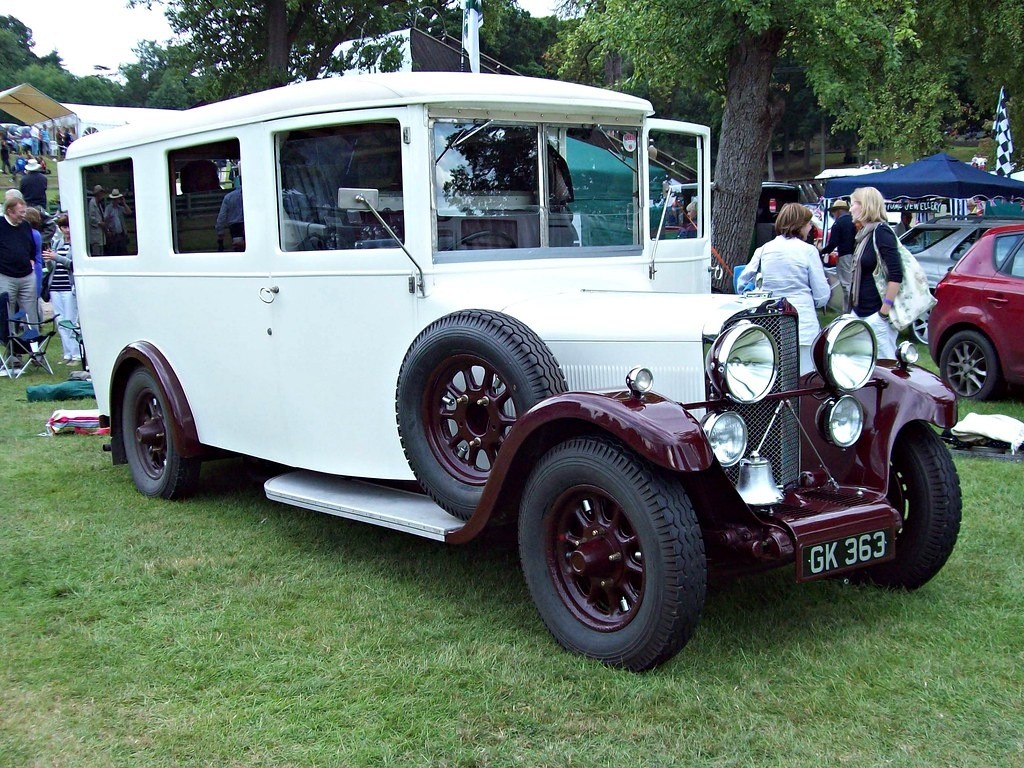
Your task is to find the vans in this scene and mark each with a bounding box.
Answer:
[56,71,964,674]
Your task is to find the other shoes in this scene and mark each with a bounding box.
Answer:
[57,358,70,365]
[8,361,21,367]
[31,359,43,367]
[66,360,80,367]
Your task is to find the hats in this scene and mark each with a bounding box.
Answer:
[827,199,850,211]
[108,188,124,198]
[24,158,42,171]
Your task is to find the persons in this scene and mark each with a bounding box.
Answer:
[818,200,857,313]
[894,211,912,238]
[736,202,830,377]
[861,154,990,170]
[87,185,132,256]
[677,202,697,239]
[847,187,904,360]
[0,124,83,369]
[215,176,245,251]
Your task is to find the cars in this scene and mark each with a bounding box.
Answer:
[927,223,1024,403]
[893,213,1024,347]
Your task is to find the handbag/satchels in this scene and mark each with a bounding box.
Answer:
[126,235,131,245]
[869,222,939,331]
[48,408,113,437]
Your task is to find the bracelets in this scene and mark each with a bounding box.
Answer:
[883,299,894,306]
[878,311,889,320]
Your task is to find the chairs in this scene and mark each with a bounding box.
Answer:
[0,291,61,380]
[59,320,89,372]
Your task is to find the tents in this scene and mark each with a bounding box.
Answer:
[823,152,1024,251]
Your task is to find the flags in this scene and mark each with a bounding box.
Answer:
[991,87,1014,178]
